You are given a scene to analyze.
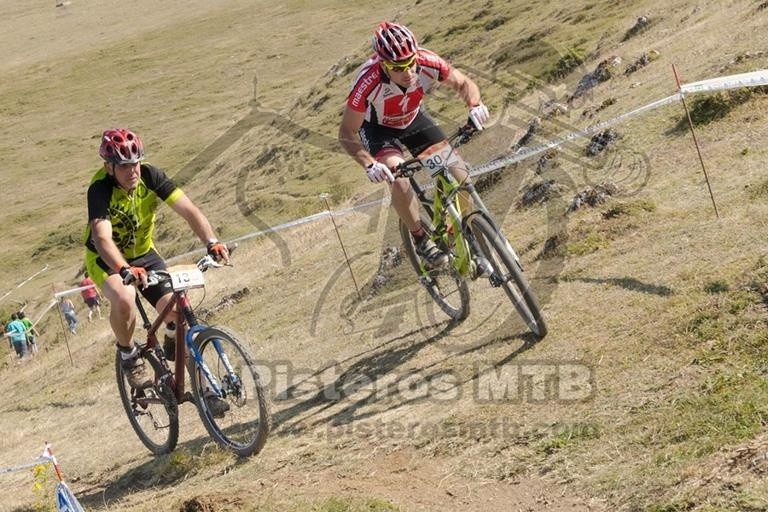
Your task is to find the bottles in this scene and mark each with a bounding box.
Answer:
[447,223,456,250]
[164,320,176,361]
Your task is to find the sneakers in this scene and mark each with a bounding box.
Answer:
[410,231,456,270]
[118,348,153,390]
[198,389,233,416]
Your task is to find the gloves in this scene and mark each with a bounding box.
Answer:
[364,162,396,185]
[465,102,492,132]
[206,240,231,265]
[116,264,149,289]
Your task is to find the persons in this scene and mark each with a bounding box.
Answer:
[80,271,105,322]
[0,311,39,358]
[59,297,79,336]
[84,128,231,390]
[338,21,489,267]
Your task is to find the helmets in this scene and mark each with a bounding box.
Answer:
[370,19,420,63]
[97,126,146,166]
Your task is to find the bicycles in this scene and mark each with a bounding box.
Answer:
[113,241,270,460]
[372,116,548,336]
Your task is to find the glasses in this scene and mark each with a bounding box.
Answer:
[381,53,419,72]
